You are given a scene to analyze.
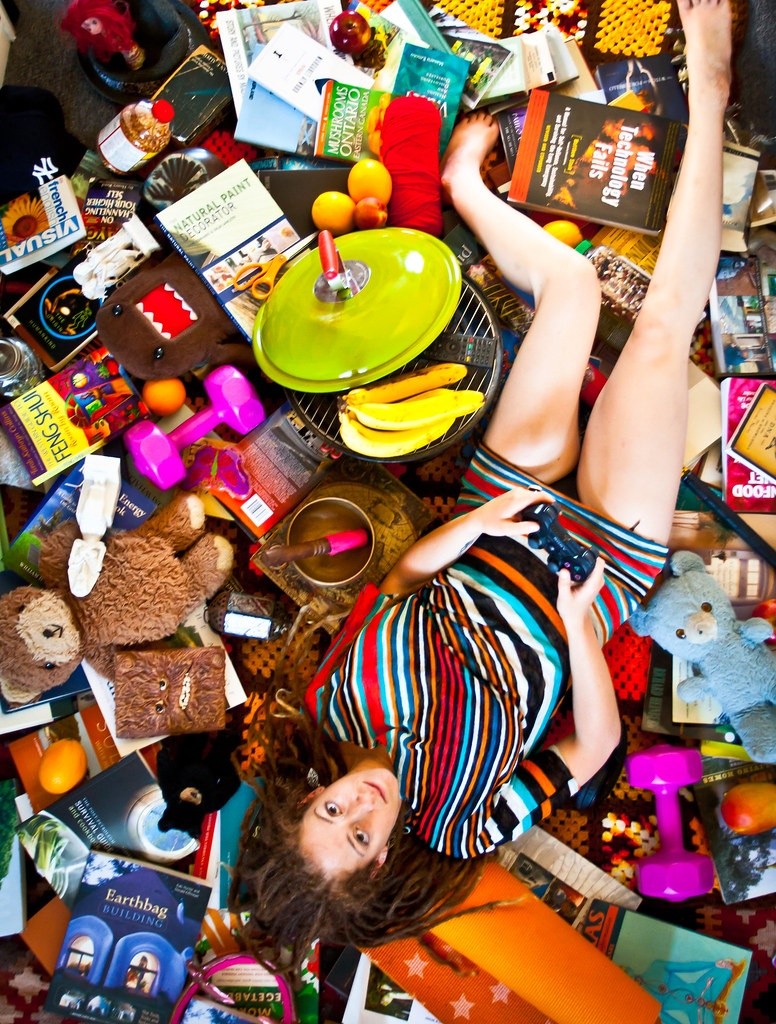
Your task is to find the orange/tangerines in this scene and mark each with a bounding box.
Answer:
[39,738,86,794]
[348,159,391,206]
[144,378,186,414]
[312,191,355,234]
[542,220,582,248]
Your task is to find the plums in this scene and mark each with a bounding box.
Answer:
[355,198,388,229]
[330,11,371,53]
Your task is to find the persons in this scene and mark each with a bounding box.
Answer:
[231,0,731,969]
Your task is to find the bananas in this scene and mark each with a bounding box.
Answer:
[337,362,486,459]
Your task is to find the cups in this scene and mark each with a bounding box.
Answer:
[284,496,376,588]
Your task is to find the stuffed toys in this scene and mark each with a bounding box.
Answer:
[96,250,237,381]
[0,490,233,704]
[627,550,776,764]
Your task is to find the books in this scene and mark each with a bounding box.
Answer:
[215,0,690,235]
[68,178,144,261]
[194,777,263,911]
[19,894,241,978]
[7,704,162,815]
[667,130,758,239]
[0,174,87,277]
[0,457,157,590]
[709,256,776,514]
[207,401,347,543]
[15,749,201,913]
[0,346,152,487]
[325,947,442,1023]
[0,778,27,937]
[174,437,239,523]
[691,750,776,905]
[493,825,754,1024]
[42,850,212,1024]
[153,159,312,343]
[151,44,232,143]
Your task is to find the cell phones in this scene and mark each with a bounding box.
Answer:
[227,594,273,619]
[222,611,271,639]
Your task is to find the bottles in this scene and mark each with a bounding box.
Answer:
[95,98,176,177]
[0,337,42,398]
[573,239,707,323]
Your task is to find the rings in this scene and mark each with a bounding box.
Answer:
[528,485,542,492]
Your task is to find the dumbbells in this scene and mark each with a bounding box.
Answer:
[627,744,715,903]
[125,365,266,490]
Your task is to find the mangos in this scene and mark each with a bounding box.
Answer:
[721,782,776,834]
